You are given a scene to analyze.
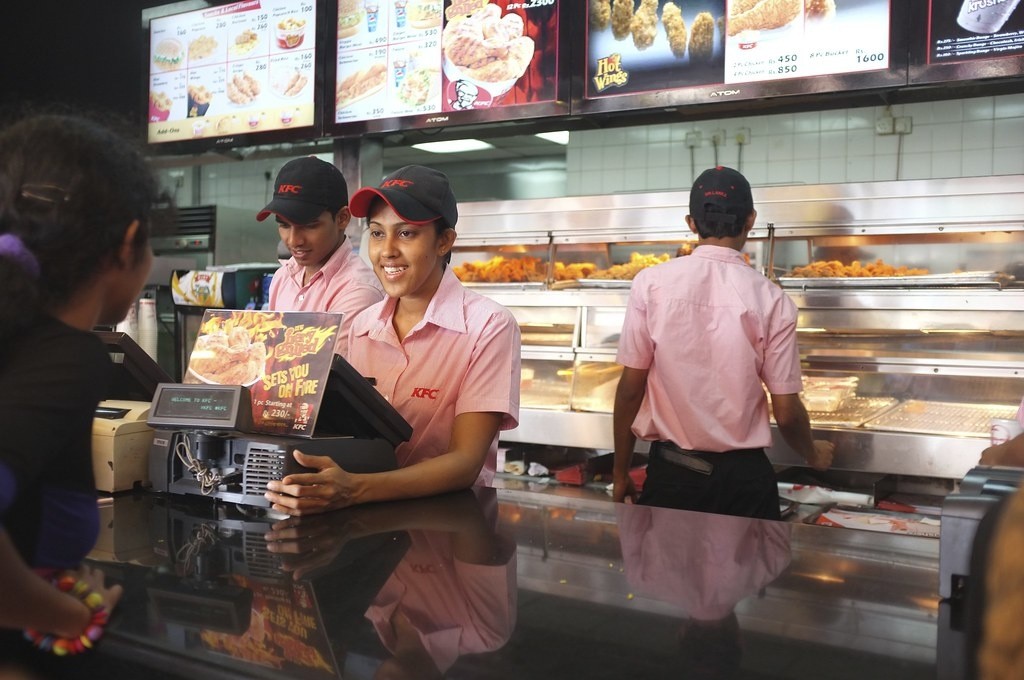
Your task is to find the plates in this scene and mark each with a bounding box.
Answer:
[188,335,266,387]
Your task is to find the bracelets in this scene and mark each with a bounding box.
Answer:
[22,567,109,655]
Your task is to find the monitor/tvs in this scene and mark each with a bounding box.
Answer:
[92,331,177,400]
[323,352,413,446]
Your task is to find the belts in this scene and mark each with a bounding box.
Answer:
[656,446,714,475]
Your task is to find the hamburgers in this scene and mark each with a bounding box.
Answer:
[407,0,443,29]
[152,41,182,71]
[337,0,363,39]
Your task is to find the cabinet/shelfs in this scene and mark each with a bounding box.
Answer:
[357,174,1024,481]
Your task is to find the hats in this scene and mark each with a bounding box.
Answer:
[689,166,753,225]
[348,164,458,227]
[257,156,347,225]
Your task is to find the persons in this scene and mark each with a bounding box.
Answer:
[264,166,521,517]
[0,115,174,656]
[613,166,835,521]
[613,503,792,675]
[257,157,386,358]
[263,486,519,679]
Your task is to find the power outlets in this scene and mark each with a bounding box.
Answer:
[173,176,184,187]
[684,130,703,149]
[710,128,726,146]
[894,116,912,134]
[735,126,751,145]
[876,116,894,135]
[263,166,276,180]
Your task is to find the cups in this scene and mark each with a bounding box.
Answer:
[957,0,1019,33]
[109,299,158,364]
[366,6,378,32]
[393,61,406,87]
[394,1,406,27]
